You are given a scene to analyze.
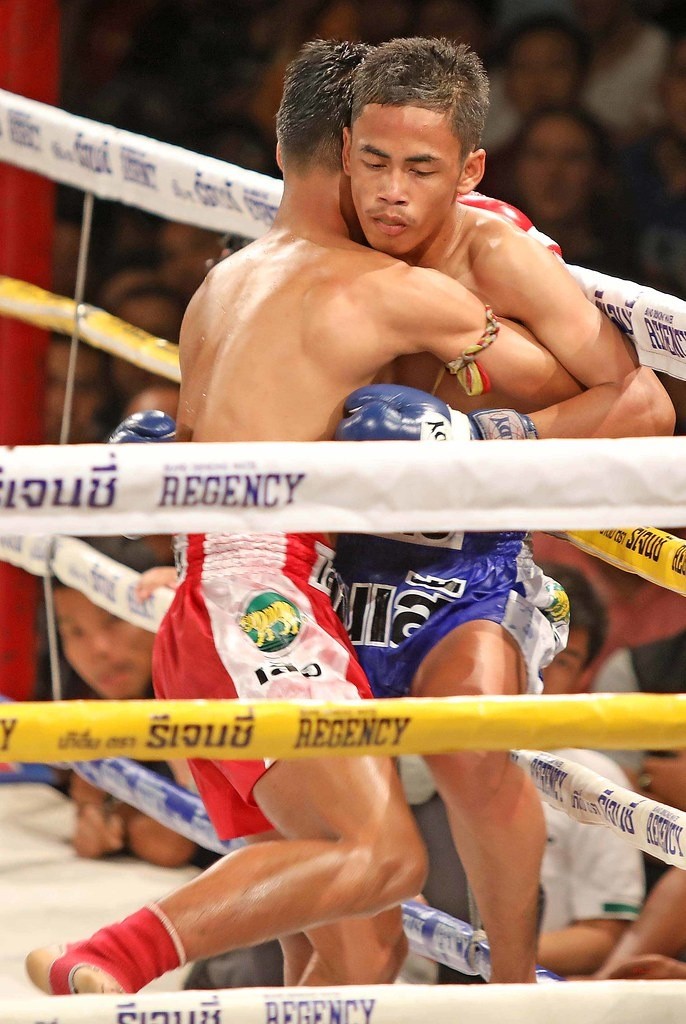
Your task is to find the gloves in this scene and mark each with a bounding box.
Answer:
[456,191,562,258]
[332,384,538,543]
[107,410,176,541]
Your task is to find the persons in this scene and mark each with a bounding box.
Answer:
[28,0,686,999]
